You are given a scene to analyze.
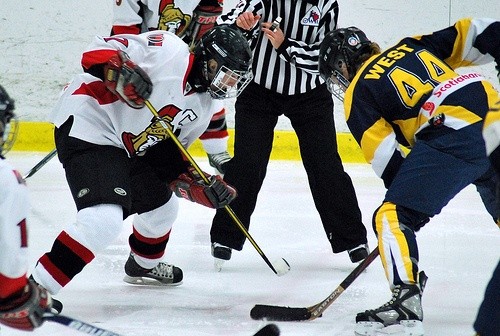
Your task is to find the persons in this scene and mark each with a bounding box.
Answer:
[210,0,371,272]
[0,84,52,332]
[318,17,500,336]
[27,0,237,313]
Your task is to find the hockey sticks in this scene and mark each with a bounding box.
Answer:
[145,99,291,278]
[250,246,380,322]
[22,147,58,180]
[41,310,120,336]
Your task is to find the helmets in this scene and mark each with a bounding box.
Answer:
[193,26,252,76]
[317,27,372,83]
[0,84,17,159]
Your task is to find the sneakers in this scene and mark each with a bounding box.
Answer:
[348,241,371,273]
[352,281,427,336]
[124,253,184,289]
[211,241,232,272]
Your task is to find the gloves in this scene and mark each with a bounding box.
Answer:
[0,279,54,332]
[175,173,238,209]
[103,49,154,112]
